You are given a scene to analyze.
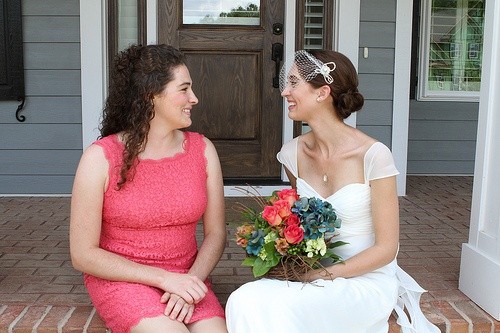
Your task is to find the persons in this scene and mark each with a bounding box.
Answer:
[70,44,229,332]
[226,48,399,333]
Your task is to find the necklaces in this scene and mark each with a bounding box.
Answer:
[315,149,333,183]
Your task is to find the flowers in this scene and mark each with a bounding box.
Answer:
[228,183,349,289]
